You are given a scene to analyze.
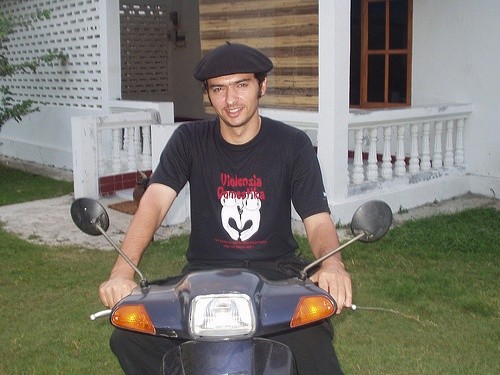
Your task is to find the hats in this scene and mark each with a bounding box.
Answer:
[193,44,273,81]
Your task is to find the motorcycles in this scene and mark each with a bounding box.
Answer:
[69,197,392,375]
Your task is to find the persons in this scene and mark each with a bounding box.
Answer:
[98,44,353,374]
